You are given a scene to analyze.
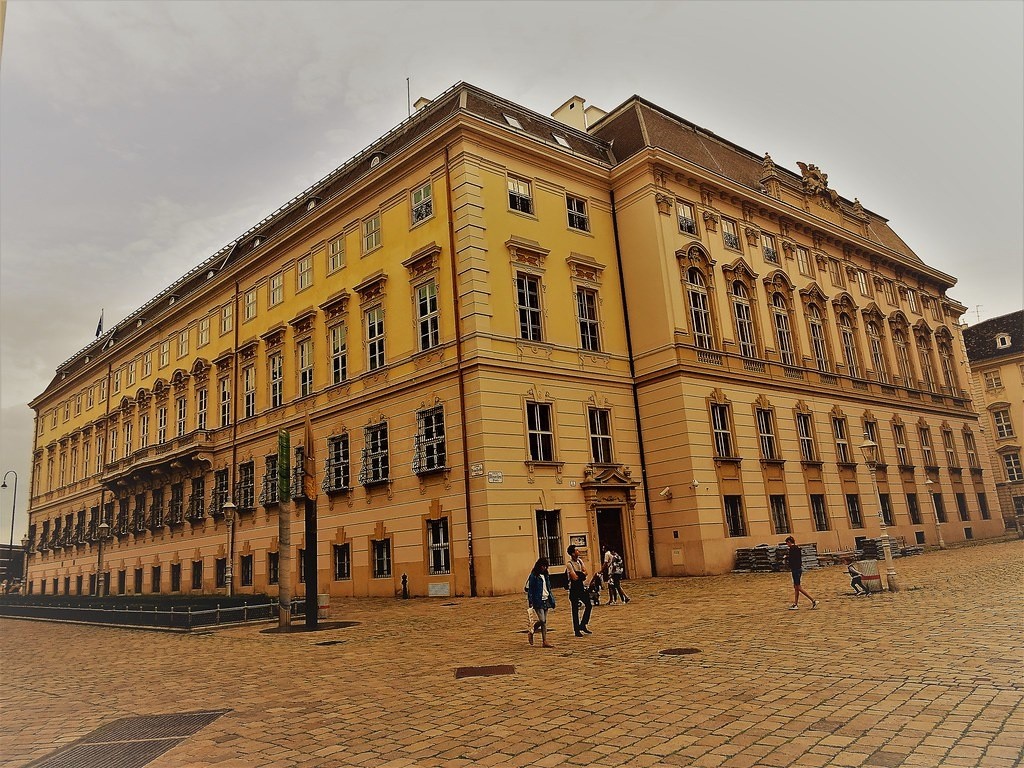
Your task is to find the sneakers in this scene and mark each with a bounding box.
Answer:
[867,592,871,596]
[857,592,861,595]
[621,602,625,605]
[606,601,620,605]
[789,604,798,609]
[812,600,819,609]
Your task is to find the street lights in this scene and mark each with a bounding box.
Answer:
[98,519,110,597]
[1,470,19,595]
[1005,477,1024,539]
[925,475,946,550]
[221,496,236,597]
[857,431,899,591]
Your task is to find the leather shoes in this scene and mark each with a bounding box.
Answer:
[585,631,591,633]
[542,644,554,648]
[575,632,583,637]
[528,632,533,645]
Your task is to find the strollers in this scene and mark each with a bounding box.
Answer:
[577,572,604,607]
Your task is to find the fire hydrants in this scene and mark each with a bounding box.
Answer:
[401,572,410,600]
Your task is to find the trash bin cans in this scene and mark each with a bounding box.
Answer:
[857,559,882,592]
[317,594,330,619]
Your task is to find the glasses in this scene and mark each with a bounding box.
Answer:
[543,565,548,567]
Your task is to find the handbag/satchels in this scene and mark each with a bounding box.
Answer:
[524,587,529,592]
[527,607,540,629]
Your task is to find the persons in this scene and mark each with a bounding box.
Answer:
[601,546,625,605]
[521,558,554,648]
[566,545,592,637]
[782,536,820,611]
[840,558,872,597]
[598,545,630,606]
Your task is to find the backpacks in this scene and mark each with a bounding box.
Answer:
[563,571,569,590]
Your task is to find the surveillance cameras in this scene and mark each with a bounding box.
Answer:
[693,482,699,487]
[660,487,669,496]
